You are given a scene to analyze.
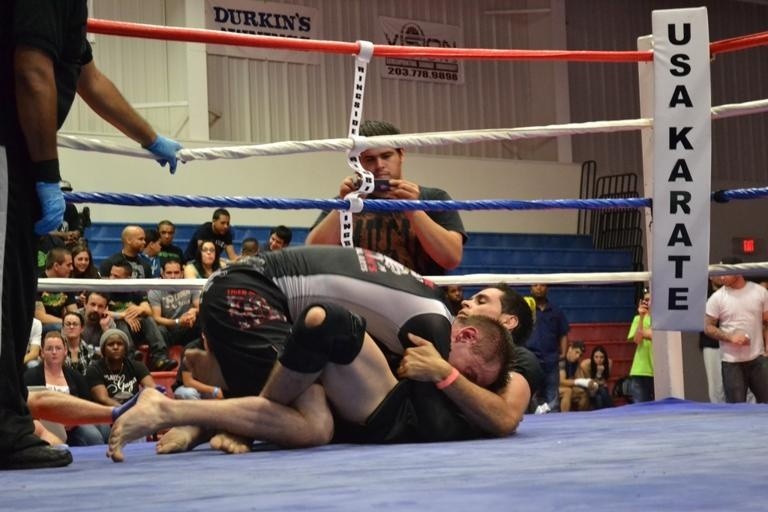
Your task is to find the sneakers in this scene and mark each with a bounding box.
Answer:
[150,352,178,371]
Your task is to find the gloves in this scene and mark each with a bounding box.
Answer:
[112,385,167,423]
[142,133,185,174]
[35,182,66,237]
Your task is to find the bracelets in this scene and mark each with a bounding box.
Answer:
[436,368,458,389]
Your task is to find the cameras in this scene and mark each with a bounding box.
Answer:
[354,179,394,191]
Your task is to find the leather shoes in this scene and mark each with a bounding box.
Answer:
[0,444,73,470]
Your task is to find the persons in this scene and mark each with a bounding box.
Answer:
[105,245,516,463]
[699,258,767,404]
[1,0,185,472]
[26,181,294,444]
[304,120,469,273]
[210,282,546,454]
[523,283,570,416]
[581,346,615,408]
[557,340,598,413]
[627,287,656,403]
[444,284,465,306]
